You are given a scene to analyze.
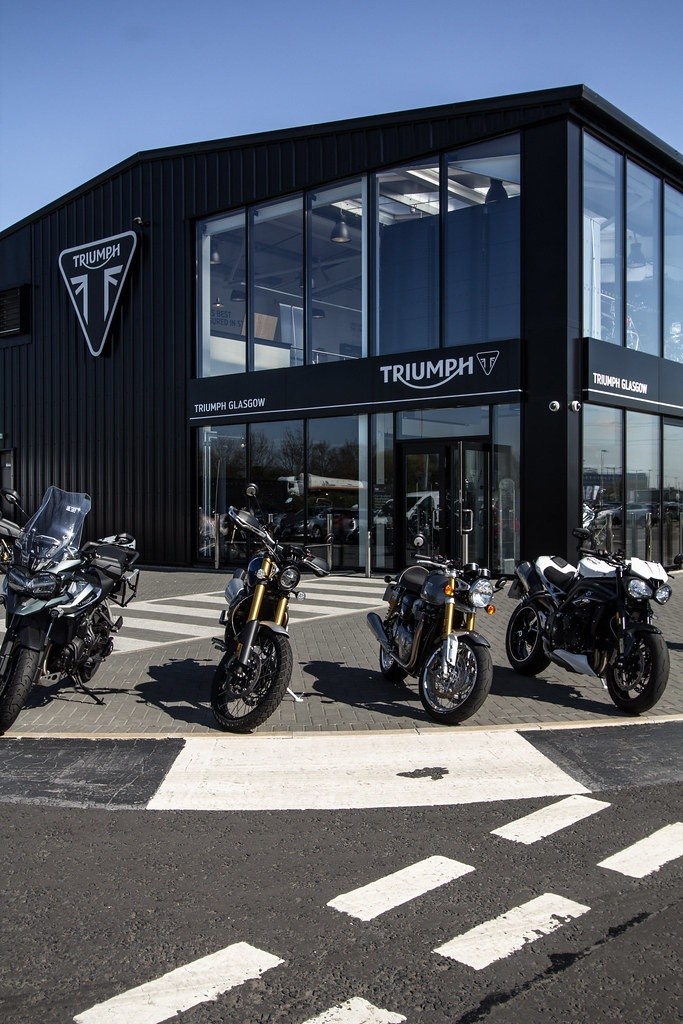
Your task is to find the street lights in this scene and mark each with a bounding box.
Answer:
[599,449,609,513]
[630,469,644,505]
[647,468,655,494]
[604,466,622,504]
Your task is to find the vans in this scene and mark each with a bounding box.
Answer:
[373,490,441,541]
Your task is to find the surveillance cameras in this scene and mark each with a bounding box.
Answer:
[572,400,581,412]
[549,401,560,411]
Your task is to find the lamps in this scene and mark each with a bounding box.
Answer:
[209,247,222,264]
[329,208,351,243]
[230,290,245,301]
[626,232,646,267]
[313,309,324,318]
[213,296,224,309]
[484,178,508,204]
[300,276,315,288]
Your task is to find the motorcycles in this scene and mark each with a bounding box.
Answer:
[365,532,509,725]
[209,483,336,734]
[0,484,142,734]
[505,527,675,714]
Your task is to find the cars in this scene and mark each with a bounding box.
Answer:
[262,504,361,543]
[582,499,683,527]
